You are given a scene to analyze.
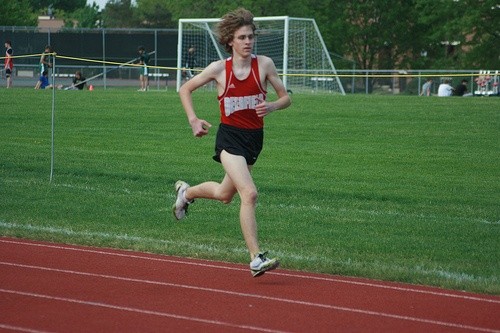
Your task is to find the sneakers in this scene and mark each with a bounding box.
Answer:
[249,249,282,277]
[172,179,194,220]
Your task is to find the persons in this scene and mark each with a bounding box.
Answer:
[173,8,291,278]
[137,46,152,91]
[185,45,197,80]
[34,46,93,91]
[420,74,472,98]
[3,40,14,88]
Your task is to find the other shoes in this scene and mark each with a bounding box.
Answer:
[137,87,149,91]
